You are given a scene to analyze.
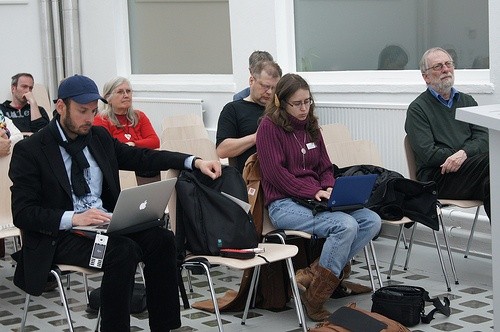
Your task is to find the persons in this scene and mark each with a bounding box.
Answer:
[445,48,458,69]
[89,75,161,151]
[377,45,409,69]
[471,56,489,69]
[255,73,381,322]
[231,51,274,102]
[0,114,23,258]
[6,74,221,332]
[404,47,491,227]
[216,60,283,178]
[0,73,51,132]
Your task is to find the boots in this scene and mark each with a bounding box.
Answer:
[295,258,351,322]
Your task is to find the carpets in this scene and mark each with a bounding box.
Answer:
[0,236,494,332]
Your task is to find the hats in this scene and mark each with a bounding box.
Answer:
[58,74,109,104]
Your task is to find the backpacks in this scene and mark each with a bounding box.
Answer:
[173,166,256,261]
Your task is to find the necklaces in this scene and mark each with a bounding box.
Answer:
[288,120,308,171]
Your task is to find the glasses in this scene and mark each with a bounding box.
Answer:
[426,61,454,69]
[254,80,277,92]
[288,99,313,107]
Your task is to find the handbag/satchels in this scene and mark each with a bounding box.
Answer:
[373,282,451,326]
[86,284,149,314]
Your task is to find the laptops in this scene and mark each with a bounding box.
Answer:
[72,178,178,233]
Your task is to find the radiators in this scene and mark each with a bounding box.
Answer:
[313,102,418,180]
[132,98,206,126]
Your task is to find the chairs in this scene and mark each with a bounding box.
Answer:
[0,114,488,332]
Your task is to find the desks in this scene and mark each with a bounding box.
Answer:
[455,106,500,332]
